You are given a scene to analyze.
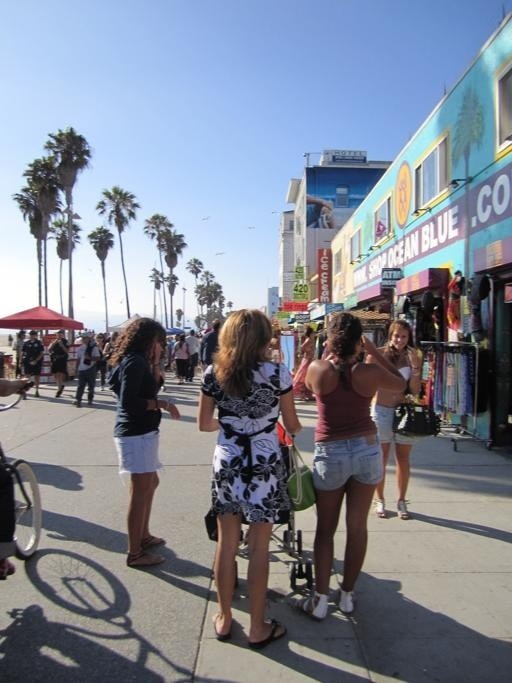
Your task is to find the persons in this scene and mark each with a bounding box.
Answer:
[196,309,301,651]
[107,316,182,566]
[366,319,424,521]
[289,310,406,620]
[14,318,321,405]
[1,373,30,580]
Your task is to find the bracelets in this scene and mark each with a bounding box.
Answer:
[411,372,420,376]
[165,402,169,411]
[410,366,419,370]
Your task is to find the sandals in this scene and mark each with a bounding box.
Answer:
[288,592,328,621]
[375,500,386,519]
[396,500,411,520]
[335,590,355,614]
[127,534,167,567]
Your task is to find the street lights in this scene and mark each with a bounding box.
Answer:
[182,286,188,332]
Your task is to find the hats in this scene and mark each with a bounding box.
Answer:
[77,332,88,338]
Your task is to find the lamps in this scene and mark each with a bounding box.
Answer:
[411,207,431,215]
[447,176,473,188]
[349,245,380,265]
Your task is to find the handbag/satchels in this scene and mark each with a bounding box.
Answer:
[285,444,317,511]
[392,402,441,439]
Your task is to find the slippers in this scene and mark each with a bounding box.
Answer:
[213,613,232,641]
[248,618,287,651]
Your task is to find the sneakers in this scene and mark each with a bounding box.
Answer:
[72,401,81,406]
[0,557,16,581]
[55,385,64,398]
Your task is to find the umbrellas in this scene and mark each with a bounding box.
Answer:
[0,304,82,329]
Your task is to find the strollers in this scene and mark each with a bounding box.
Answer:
[229,427,313,592]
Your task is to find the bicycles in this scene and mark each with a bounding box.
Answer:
[0,379,45,563]
